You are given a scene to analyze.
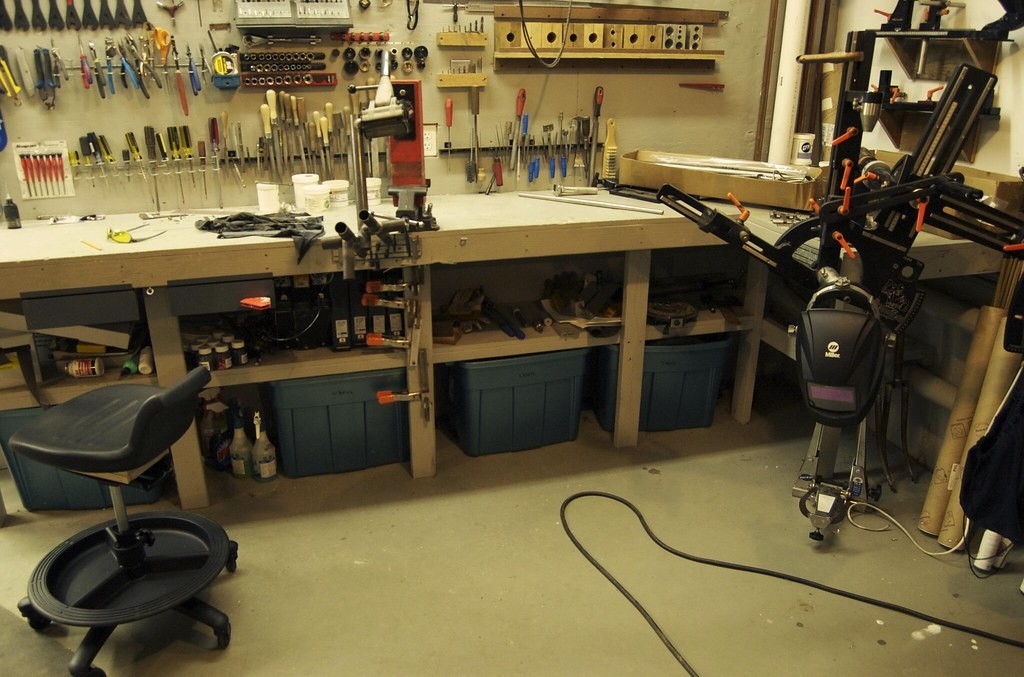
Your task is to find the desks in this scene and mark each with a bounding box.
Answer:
[0,185,1024,557]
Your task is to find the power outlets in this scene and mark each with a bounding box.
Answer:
[423,124,437,157]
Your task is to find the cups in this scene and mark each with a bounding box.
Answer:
[257,182,279,212]
[791,134,815,165]
[323,180,349,207]
[305,184,330,213]
[366,178,382,205]
[292,174,319,209]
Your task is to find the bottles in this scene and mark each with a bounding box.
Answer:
[181,325,247,372]
[120,355,139,375]
[4,199,21,229]
[138,346,153,374]
[64,357,105,378]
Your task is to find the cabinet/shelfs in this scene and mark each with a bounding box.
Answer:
[865,26,1015,162]
[491,5,728,58]
[436,32,488,86]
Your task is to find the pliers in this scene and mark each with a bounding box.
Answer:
[3,30,213,118]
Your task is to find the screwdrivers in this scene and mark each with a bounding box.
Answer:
[14,79,610,207]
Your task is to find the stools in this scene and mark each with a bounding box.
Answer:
[7,365,238,677]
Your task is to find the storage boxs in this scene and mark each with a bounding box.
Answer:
[620,150,824,214]
[439,347,600,457]
[264,369,411,477]
[0,405,175,512]
[595,332,739,432]
